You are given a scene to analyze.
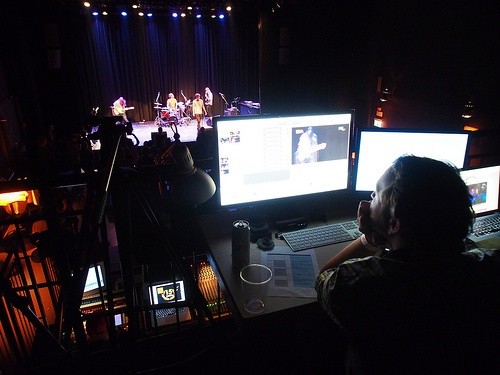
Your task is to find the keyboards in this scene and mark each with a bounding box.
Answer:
[281,219,364,252]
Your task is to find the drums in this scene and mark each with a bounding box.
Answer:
[167,115,178,125]
[160,109,169,119]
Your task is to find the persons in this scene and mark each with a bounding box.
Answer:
[192,93,207,130]
[167,93,177,114]
[113,97,129,126]
[204,87,213,119]
[295,127,319,164]
[314,156,500,375]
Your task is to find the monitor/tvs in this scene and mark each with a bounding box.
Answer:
[353,126,470,201]
[213,106,357,225]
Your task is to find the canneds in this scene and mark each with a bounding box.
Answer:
[232,220,250,270]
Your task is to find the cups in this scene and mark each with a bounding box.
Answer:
[239,264,273,314]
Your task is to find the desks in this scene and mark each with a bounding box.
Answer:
[196,195,500,324]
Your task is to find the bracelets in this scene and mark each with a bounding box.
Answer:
[361,234,380,252]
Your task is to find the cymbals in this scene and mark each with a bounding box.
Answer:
[154,107,161,108]
[153,103,162,105]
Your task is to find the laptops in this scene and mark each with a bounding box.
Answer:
[456,164,500,244]
[148,280,192,327]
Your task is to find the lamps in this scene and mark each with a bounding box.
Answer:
[129,145,217,324]
